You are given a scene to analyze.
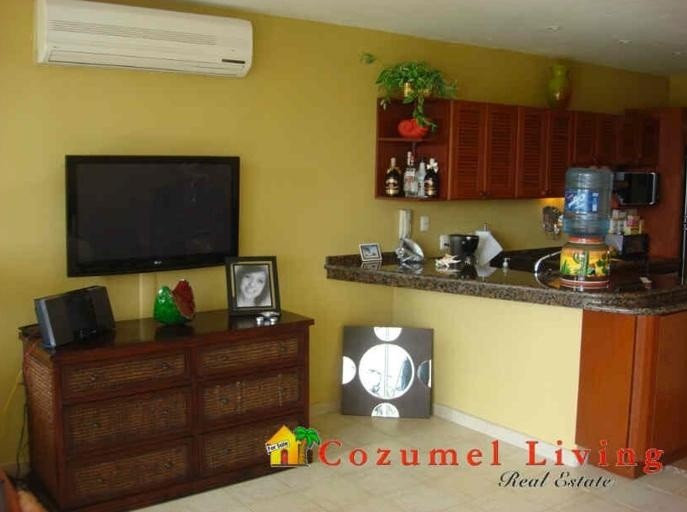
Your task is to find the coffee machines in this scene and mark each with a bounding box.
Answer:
[449,234,479,268]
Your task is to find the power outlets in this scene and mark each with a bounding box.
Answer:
[440,234,450,249]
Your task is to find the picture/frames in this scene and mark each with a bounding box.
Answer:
[225,256,281,317]
[359,242,383,261]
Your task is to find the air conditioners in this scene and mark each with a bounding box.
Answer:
[34,0,254,78]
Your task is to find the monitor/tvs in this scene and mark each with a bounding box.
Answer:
[64,153,241,277]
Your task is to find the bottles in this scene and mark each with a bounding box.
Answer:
[501,258,511,267]
[545,64,573,111]
[385,150,439,197]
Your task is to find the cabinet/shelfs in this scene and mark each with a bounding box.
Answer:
[621,105,687,273]
[373,98,658,202]
[17,307,315,512]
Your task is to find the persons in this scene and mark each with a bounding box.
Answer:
[235,265,269,309]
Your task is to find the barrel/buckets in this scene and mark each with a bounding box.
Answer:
[561,167,614,236]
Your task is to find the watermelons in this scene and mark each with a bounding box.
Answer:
[153,279,195,325]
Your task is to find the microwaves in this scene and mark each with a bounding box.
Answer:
[618,171,657,206]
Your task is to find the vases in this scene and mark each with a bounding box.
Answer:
[547,65,573,108]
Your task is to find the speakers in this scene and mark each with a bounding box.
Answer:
[34,286,114,348]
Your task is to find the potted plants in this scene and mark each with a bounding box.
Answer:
[379,62,460,131]
